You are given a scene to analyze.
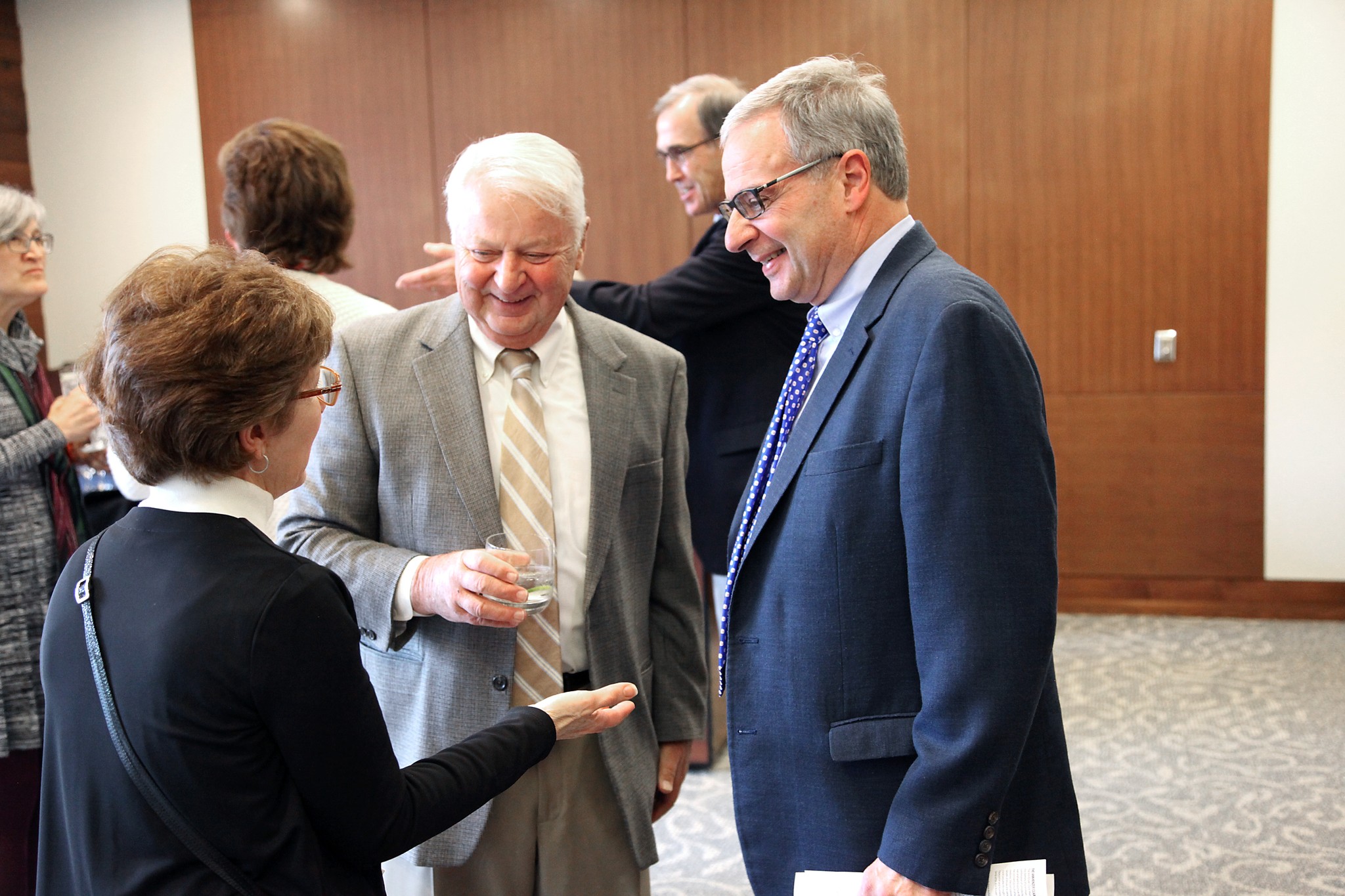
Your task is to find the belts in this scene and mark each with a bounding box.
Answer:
[563,671,593,693]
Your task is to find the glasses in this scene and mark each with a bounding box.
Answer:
[287,366,342,406]
[652,133,721,168]
[717,152,846,219]
[7,230,55,254]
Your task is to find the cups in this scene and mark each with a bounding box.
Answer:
[483,533,555,616]
[59,371,106,453]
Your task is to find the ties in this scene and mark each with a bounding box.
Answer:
[497,347,564,707]
[719,310,826,698]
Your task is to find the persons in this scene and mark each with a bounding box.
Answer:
[107,117,399,502]
[0,186,102,896]
[719,56,1090,896]
[396,74,814,622]
[34,246,640,896]
[275,133,709,896]
